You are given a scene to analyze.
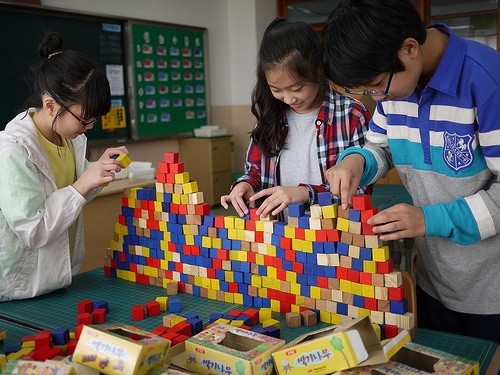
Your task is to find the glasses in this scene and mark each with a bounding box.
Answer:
[344,47,397,97]
[54,98,97,130]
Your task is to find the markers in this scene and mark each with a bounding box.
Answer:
[116,139,126,143]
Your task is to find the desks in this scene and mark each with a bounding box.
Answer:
[77,177,156,276]
[0,318,42,356]
[0,266,500,375]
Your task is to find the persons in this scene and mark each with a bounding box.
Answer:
[0,31,130,302]
[221,15,374,220]
[322,0,500,346]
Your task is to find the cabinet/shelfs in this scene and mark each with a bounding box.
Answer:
[177,135,233,210]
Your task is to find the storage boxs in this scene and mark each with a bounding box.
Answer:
[0,315,480,375]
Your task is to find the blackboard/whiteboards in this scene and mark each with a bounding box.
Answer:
[0,1,132,146]
